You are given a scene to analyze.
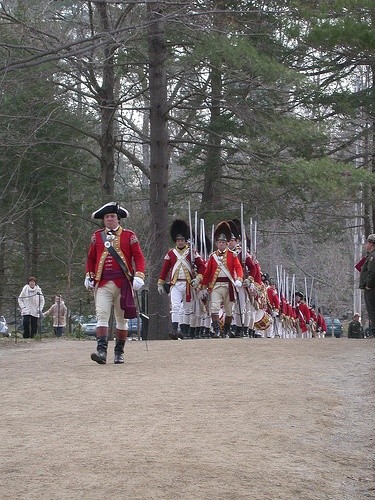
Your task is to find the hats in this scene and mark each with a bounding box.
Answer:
[353,313,362,317]
[91,202,129,220]
[289,290,320,313]
[227,221,240,239]
[214,220,232,242]
[365,234,375,242]
[232,218,241,236]
[260,270,278,294]
[169,219,189,242]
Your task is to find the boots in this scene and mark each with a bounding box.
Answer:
[113,338,126,363]
[223,315,233,340]
[229,325,270,338]
[90,336,108,364]
[169,322,211,340]
[211,315,221,338]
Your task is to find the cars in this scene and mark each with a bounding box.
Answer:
[324,317,343,338]
[71,315,97,339]
[127,317,142,337]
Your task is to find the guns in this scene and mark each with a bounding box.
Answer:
[240,200,257,279]
[211,224,215,253]
[187,200,206,279]
[276,264,323,328]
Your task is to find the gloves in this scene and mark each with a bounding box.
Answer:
[84,277,94,291]
[132,277,146,291]
[243,279,251,287]
[235,280,242,288]
[189,278,200,289]
[157,282,165,296]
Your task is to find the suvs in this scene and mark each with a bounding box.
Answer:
[0,316,10,338]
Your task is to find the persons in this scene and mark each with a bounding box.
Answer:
[17,277,45,339]
[347,313,364,339]
[42,295,67,337]
[157,219,328,340]
[84,202,146,364]
[358,234,375,338]
